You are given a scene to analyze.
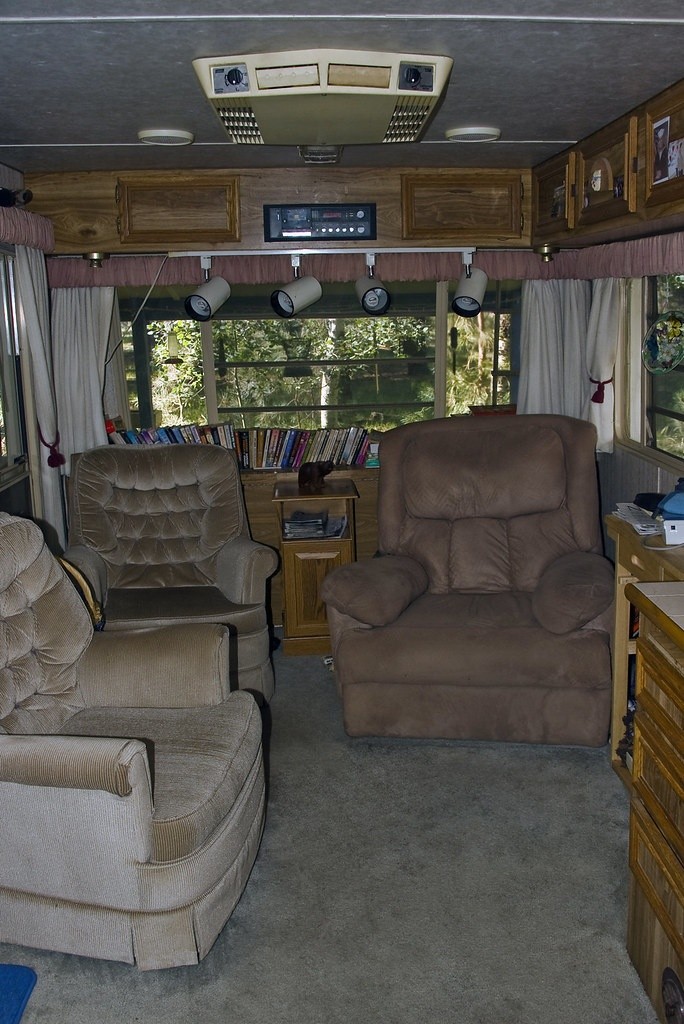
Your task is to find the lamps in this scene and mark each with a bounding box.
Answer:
[167,247,489,323]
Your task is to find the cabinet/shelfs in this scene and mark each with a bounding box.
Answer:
[625,579,684,1024]
[240,468,379,628]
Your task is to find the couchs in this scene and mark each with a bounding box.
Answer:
[319,414,615,748]
[61,443,279,708]
[0,510,267,972]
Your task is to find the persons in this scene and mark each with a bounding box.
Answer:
[653,128,670,181]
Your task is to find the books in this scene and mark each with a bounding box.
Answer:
[103,414,388,469]
[284,511,346,539]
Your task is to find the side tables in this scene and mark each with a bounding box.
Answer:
[272,480,359,656]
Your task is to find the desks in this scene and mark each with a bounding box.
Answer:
[605,513,684,795]
